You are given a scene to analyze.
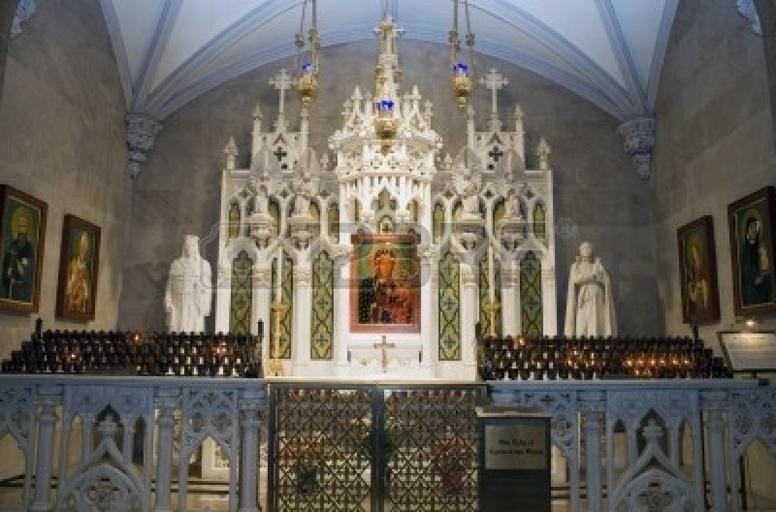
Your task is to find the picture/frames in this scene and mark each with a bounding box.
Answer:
[349,233,421,335]
[677,215,719,323]
[54,212,101,322]
[0,184,49,314]
[727,185,776,315]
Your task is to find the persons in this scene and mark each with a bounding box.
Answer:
[64,231,92,313]
[164,233,212,334]
[565,240,617,338]
[687,243,716,322]
[0,214,36,302]
[359,249,413,325]
[741,217,769,307]
